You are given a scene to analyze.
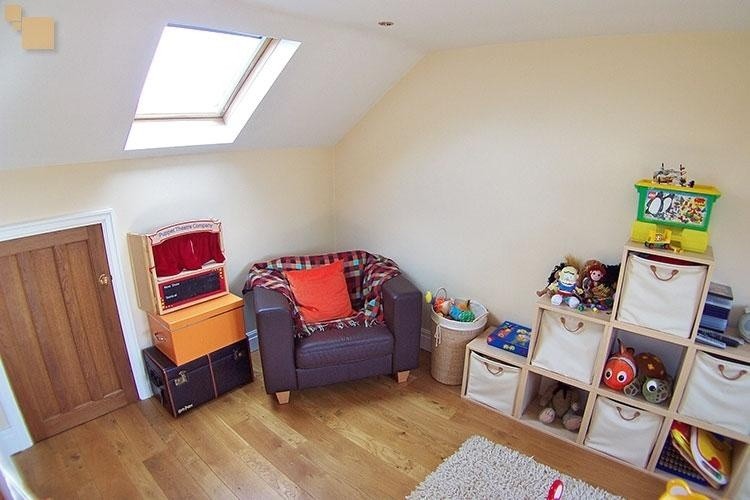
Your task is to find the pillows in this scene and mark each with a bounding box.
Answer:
[285,258,354,325]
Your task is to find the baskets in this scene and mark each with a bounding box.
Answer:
[430,289,489,385]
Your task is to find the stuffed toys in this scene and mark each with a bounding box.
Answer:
[538,380,583,431]
[426,290,475,322]
[601,337,674,404]
[536,255,621,315]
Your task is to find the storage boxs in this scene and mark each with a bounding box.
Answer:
[141,334,255,419]
[145,292,246,366]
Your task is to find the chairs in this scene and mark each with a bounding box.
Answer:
[252,250,423,405]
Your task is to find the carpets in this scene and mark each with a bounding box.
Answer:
[405,435,625,500]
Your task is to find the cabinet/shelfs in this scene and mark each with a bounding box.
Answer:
[460,236,750,500]
